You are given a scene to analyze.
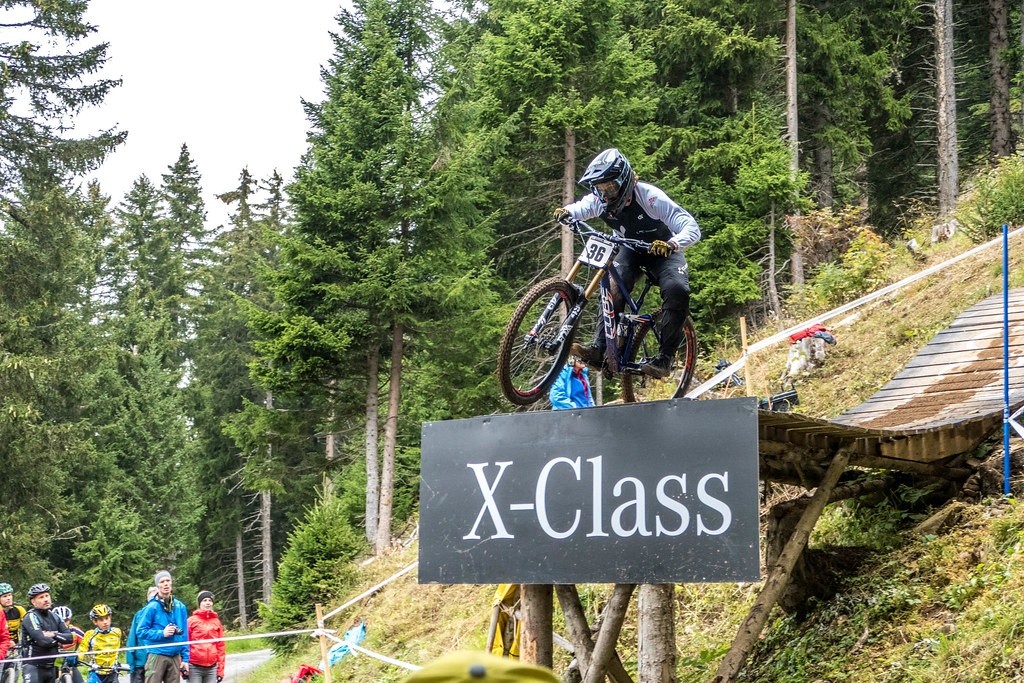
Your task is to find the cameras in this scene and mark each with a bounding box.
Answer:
[169,625,184,635]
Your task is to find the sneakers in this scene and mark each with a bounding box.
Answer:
[642,354,675,377]
[571,343,603,372]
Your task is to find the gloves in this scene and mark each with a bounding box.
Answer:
[648,240,673,257]
[182,674,190,681]
[553,207,570,223]
[217,676,222,682]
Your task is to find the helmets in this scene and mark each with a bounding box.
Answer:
[578,148,635,217]
[52,607,72,620]
[28,583,50,600]
[0,583,13,596]
[90,604,113,619]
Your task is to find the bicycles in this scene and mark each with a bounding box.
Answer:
[59,651,131,683]
[499,210,701,408]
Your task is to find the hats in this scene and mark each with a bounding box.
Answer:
[198,591,214,606]
[155,572,171,587]
[147,587,158,600]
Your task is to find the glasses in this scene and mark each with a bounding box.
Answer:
[593,179,622,197]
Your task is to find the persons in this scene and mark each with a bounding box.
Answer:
[65,604,122,683]
[549,344,594,409]
[52,606,84,683]
[136,571,189,683]
[22,583,74,683]
[125,587,158,683]
[187,591,226,683]
[554,149,701,377]
[0,583,27,683]
